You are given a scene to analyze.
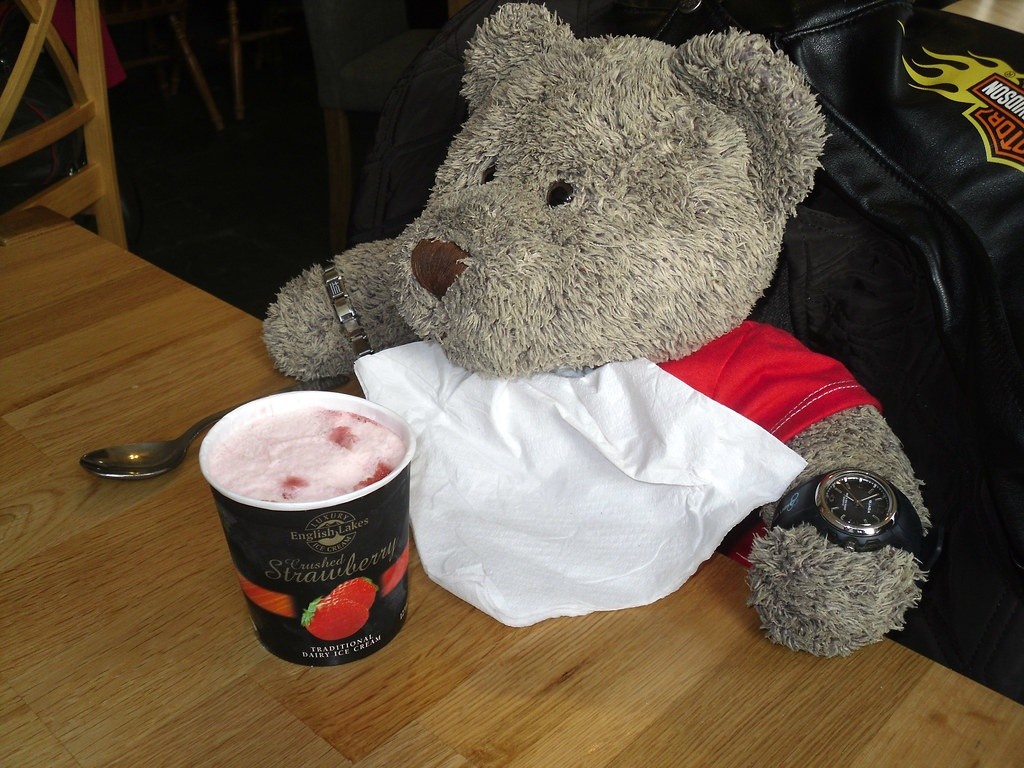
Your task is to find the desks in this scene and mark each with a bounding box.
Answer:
[0,202,1024,768]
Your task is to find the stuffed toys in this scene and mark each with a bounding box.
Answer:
[259,2,932,658]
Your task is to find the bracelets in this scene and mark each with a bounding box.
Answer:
[318,259,374,359]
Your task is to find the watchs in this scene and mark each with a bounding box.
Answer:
[772,468,929,578]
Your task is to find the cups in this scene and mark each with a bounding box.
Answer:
[199,391,417,667]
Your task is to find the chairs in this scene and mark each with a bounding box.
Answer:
[300,0,443,253]
[65,0,304,175]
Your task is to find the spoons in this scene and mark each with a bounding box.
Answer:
[80,375,350,481]
